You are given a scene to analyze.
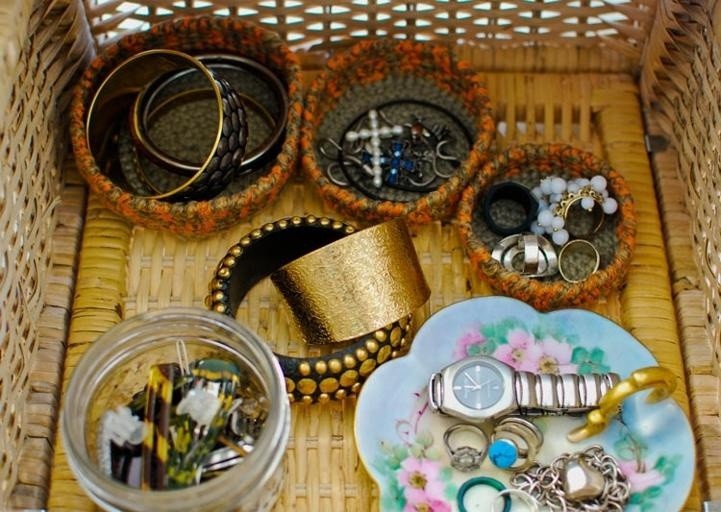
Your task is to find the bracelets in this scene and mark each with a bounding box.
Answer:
[272,217,430,345]
[208,216,413,403]
[85,49,289,202]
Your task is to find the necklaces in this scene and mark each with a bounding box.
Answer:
[518,441,631,512]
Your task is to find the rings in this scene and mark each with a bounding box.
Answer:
[443,418,544,512]
[480,168,618,285]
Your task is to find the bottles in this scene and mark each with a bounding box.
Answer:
[60,306,291,512]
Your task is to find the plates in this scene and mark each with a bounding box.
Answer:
[352,295,698,512]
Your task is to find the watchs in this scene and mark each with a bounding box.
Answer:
[426,355,619,426]
